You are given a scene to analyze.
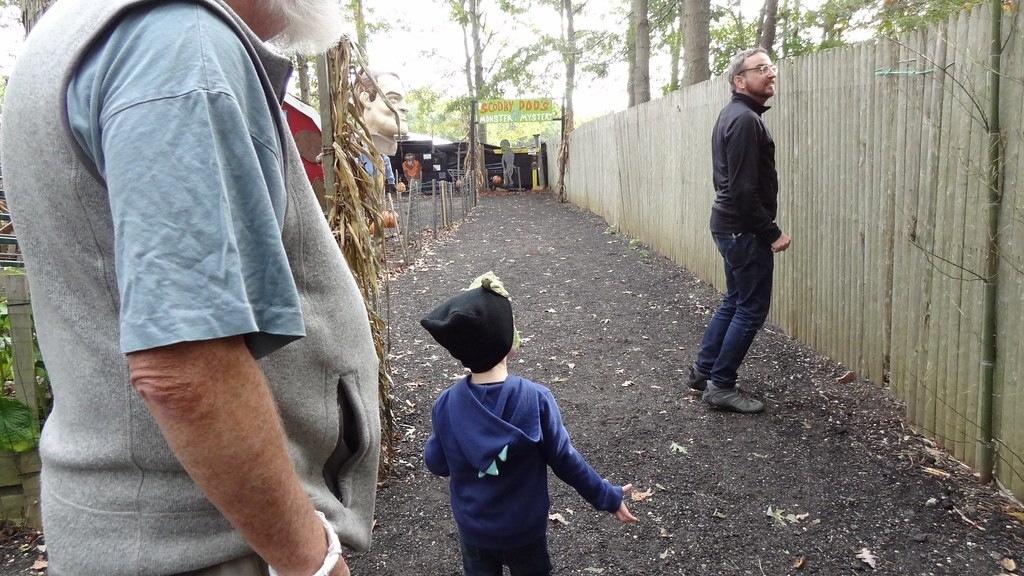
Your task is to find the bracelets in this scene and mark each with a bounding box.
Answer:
[268,509,343,576]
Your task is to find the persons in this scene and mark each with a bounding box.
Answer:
[354,71,408,155]
[417,271,640,576]
[685,47,793,414]
[0,0,381,576]
[355,145,398,211]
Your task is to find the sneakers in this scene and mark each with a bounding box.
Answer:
[702,379,764,413]
[688,364,740,391]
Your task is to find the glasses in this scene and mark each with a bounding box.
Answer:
[738,66,778,75]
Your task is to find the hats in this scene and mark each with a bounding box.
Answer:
[420,271,514,373]
